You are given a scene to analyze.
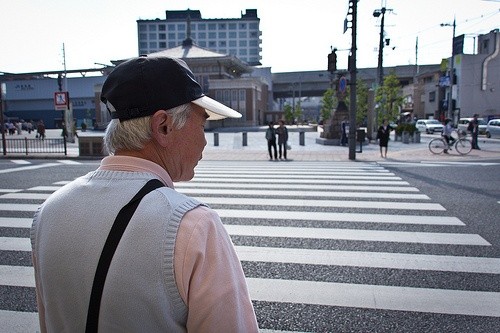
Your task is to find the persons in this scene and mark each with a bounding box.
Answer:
[30,52,260,333]
[5,119,46,142]
[467,114,481,150]
[442,118,456,154]
[265,120,288,161]
[340,116,350,146]
[375,119,390,159]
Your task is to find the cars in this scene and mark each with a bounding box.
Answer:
[485,119,500,138]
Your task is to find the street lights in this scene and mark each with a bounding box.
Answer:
[372,6,394,90]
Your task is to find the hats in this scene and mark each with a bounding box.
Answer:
[100,54,243,123]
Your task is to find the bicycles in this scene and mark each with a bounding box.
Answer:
[428,128,473,155]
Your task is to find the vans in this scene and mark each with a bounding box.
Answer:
[458,116,488,136]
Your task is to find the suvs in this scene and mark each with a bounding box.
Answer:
[415,119,445,134]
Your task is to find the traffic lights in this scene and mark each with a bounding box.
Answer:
[327,54,337,73]
